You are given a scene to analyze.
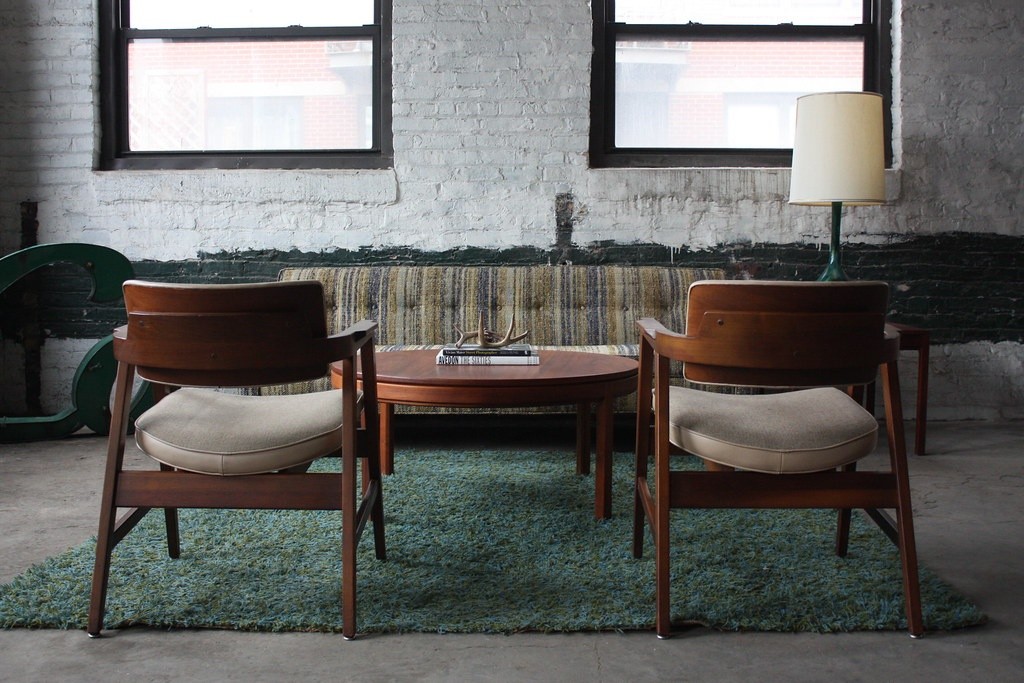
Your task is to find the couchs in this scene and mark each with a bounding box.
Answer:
[238,266,780,474]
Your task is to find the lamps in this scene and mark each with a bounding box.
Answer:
[787,92,888,280]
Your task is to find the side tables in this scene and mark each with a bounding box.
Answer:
[865,316,930,456]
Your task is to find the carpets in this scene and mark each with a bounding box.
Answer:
[0,415,991,633]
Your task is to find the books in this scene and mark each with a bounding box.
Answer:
[443,343,532,356]
[434,348,540,365]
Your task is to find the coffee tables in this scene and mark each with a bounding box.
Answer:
[329,348,639,522]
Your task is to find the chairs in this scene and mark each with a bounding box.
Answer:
[88,280,386,641]
[632,279,926,640]
